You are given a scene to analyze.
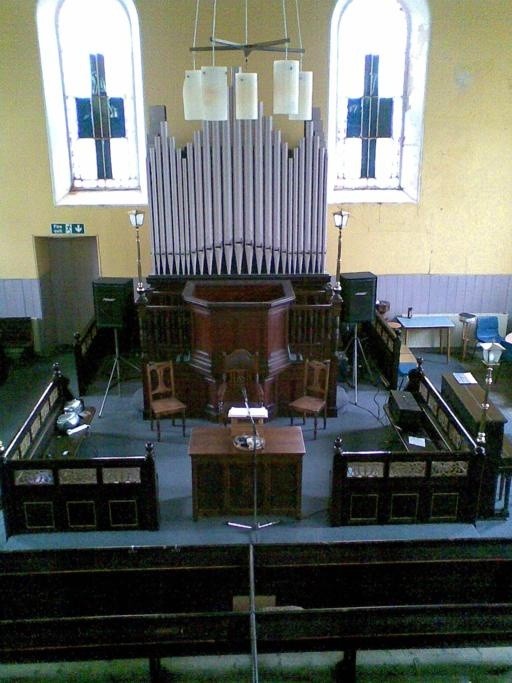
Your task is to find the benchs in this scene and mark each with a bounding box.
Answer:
[0,362,161,537]
[329,355,487,526]
[370,310,420,390]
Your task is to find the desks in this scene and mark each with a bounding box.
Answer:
[397,316,455,364]
[188,425,304,523]
[442,372,509,452]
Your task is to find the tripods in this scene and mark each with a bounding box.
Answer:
[227,400,280,544]
[335,322,377,405]
[99,329,143,419]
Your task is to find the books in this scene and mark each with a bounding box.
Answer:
[453,372,478,384]
[228,407,268,418]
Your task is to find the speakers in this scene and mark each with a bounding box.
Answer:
[340,272,377,322]
[92,277,134,329]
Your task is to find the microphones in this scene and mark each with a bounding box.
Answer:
[241,387,248,402]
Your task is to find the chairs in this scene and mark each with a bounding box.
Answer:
[288,357,332,438]
[216,350,268,426]
[469,316,512,364]
[0,316,33,368]
[143,359,186,442]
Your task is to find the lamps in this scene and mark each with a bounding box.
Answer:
[181,0,313,125]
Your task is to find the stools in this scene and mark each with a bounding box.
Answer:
[228,407,269,425]
